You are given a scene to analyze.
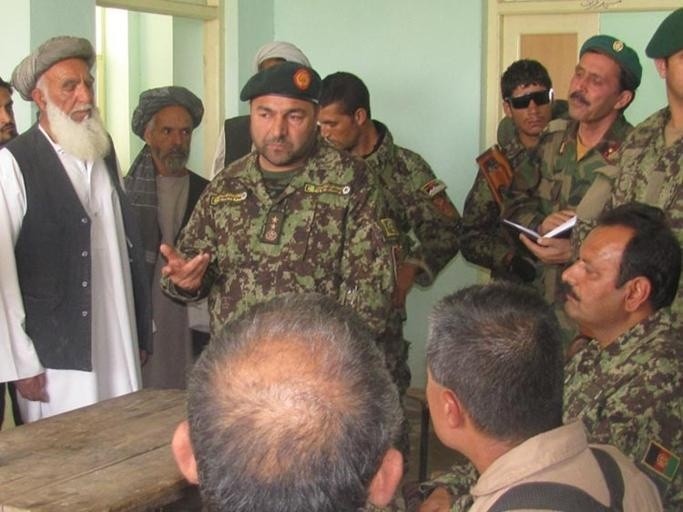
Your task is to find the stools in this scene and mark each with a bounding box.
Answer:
[404,385,432,482]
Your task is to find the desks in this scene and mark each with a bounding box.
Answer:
[0,385,205,510]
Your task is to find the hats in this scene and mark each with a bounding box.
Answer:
[577,34,643,86]
[9,35,95,102]
[644,6,683,60]
[237,60,323,110]
[129,85,204,144]
[250,39,313,82]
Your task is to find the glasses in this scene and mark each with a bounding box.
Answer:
[503,89,554,109]
